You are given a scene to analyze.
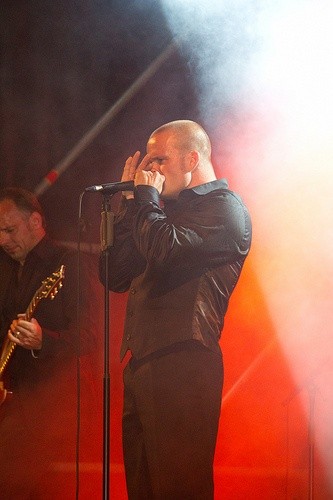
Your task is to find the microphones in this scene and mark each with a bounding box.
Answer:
[86,179,136,196]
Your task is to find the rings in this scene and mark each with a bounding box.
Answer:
[15,331,20,337]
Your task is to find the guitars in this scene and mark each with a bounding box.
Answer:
[1,264,66,405]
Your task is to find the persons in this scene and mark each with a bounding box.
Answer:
[0,186,82,401]
[95,121,252,500]
